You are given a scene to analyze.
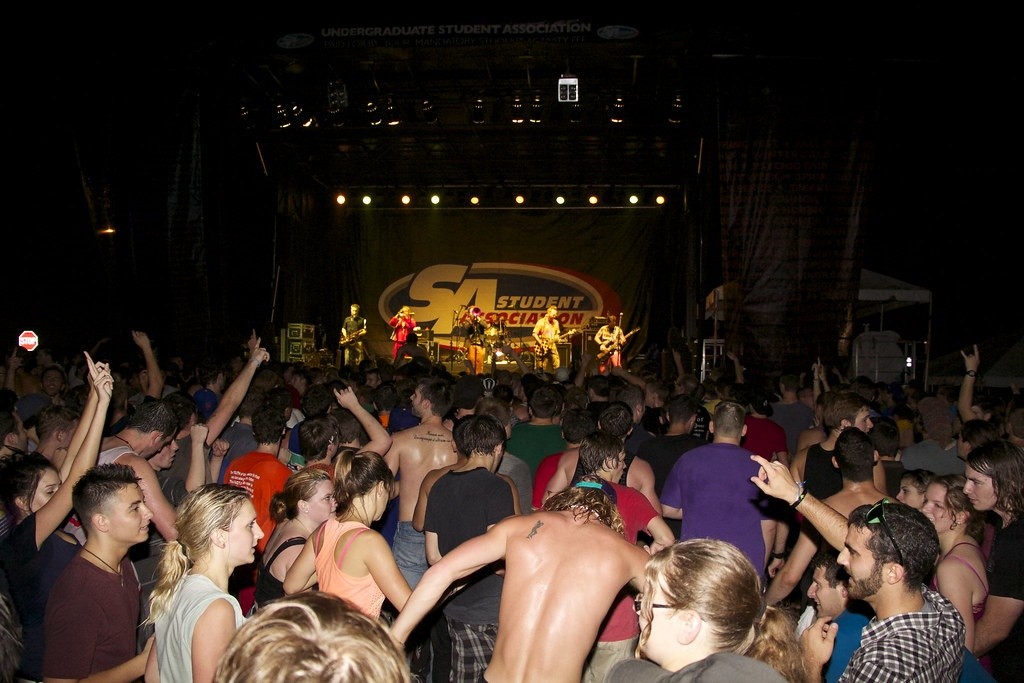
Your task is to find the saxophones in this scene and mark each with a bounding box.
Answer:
[338,329,363,345]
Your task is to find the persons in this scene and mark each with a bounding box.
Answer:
[0,329,1024,683]
[387,305,417,361]
[462,308,484,373]
[594,315,626,376]
[339,303,368,366]
[531,305,561,370]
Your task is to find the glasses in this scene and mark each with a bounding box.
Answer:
[866,497,903,567]
[634,594,706,623]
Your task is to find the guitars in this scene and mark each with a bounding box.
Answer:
[595,326,640,362]
[534,328,576,358]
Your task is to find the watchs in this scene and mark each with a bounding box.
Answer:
[967,369,977,378]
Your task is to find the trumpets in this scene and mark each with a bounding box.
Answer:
[470,306,482,317]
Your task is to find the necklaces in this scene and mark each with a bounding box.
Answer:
[81,545,127,587]
[115,435,134,451]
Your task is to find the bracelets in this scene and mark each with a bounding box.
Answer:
[814,378,819,381]
[790,482,808,509]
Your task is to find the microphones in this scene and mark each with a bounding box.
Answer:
[454,310,458,314]
[460,305,466,308]
[619,312,624,321]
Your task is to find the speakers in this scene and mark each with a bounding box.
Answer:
[544,343,572,370]
[582,331,603,377]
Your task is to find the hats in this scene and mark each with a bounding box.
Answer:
[571,474,617,504]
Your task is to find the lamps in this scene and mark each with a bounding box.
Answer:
[227,56,687,126]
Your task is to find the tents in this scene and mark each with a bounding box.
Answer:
[705,258,933,390]
